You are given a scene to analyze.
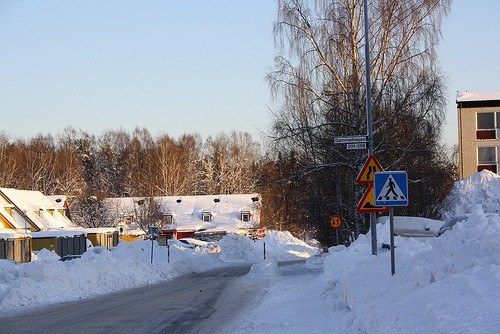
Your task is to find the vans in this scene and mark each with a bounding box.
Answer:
[438,212,499,236]
[377,216,444,237]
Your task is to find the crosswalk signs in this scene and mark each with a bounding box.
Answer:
[373,171,409,207]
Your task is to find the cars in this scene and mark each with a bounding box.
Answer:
[58,255,81,261]
[174,235,220,252]
